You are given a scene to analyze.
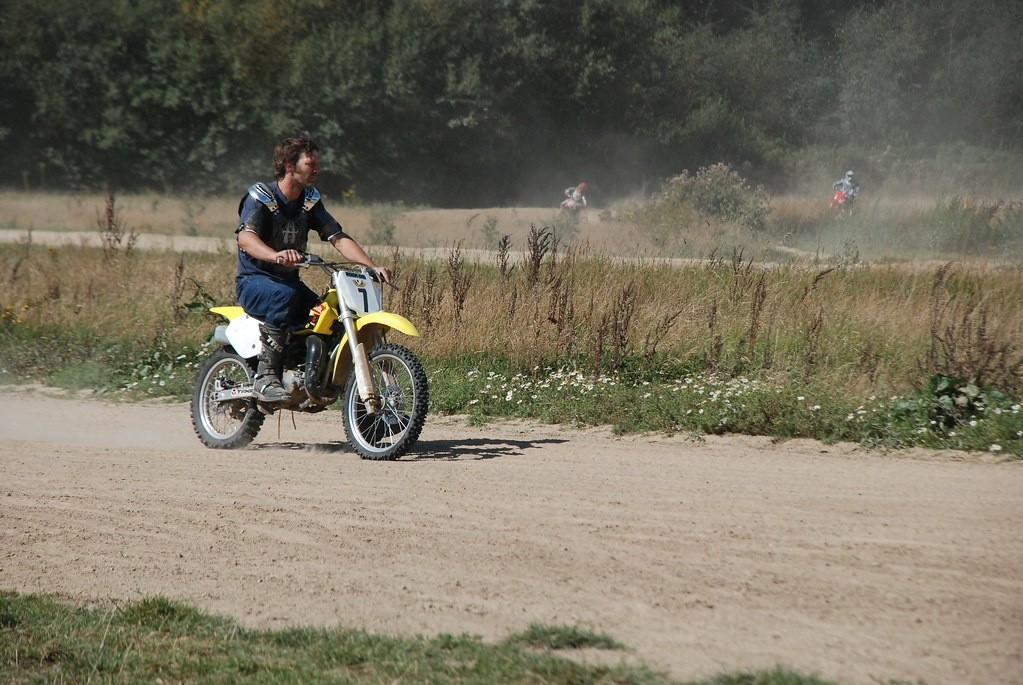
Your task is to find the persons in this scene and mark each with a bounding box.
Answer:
[560,182,587,208]
[832,170,857,212]
[234,137,391,403]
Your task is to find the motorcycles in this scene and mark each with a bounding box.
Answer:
[187,251,429,461]
[829,185,856,211]
[559,191,587,222]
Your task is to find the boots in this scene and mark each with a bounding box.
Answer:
[357,396,409,445]
[252,322,292,403]
